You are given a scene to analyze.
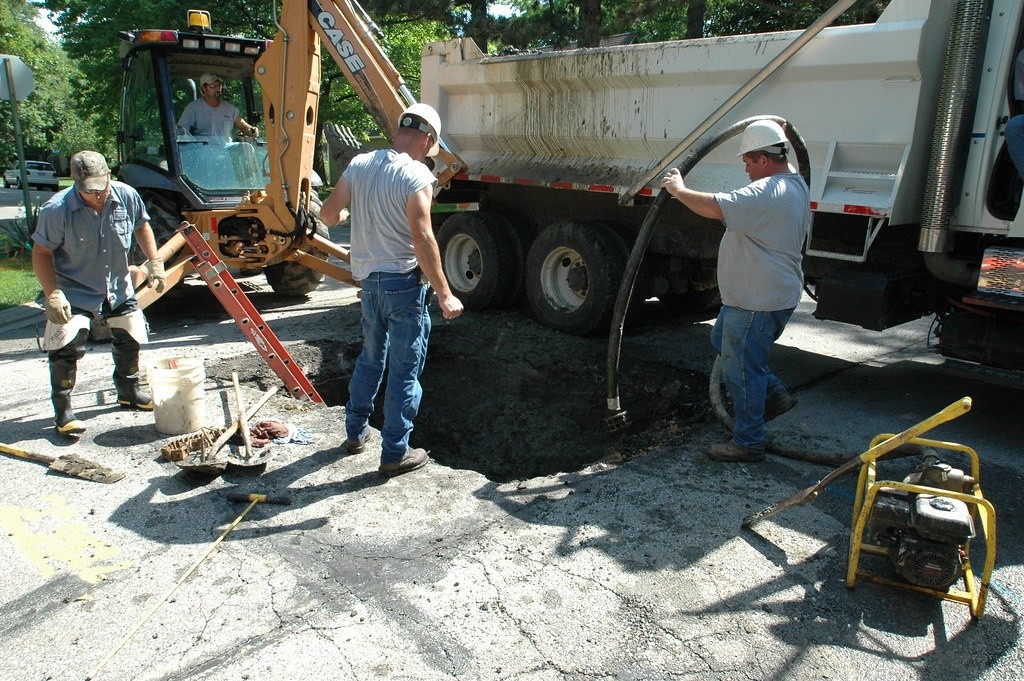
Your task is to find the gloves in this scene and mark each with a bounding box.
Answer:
[145,257,167,293]
[46,290,72,325]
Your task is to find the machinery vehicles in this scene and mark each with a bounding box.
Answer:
[110,0,469,299]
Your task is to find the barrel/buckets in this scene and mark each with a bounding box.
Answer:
[146,355,207,434]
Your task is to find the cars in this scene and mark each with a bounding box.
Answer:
[3,159,59,191]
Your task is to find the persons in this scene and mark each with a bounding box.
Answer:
[30,150,166,434]
[178,73,260,187]
[659,120,810,461]
[319,103,462,479]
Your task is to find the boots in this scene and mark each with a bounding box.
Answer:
[51,389,86,435]
[114,376,153,409]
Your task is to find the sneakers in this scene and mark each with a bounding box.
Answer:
[345,425,370,454]
[709,440,765,462]
[764,393,797,422]
[379,448,428,476]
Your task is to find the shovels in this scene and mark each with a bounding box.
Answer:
[174,372,281,474]
[0,441,126,485]
[743,395,971,536]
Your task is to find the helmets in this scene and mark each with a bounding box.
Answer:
[735,120,789,157]
[398,101,441,157]
[199,73,223,96]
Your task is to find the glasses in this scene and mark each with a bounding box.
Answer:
[206,83,221,89]
[84,177,108,194]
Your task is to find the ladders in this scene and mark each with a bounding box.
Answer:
[182,225,325,404]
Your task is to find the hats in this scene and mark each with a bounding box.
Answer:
[70,150,110,192]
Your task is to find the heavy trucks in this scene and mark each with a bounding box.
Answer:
[420,0,1024,391]
